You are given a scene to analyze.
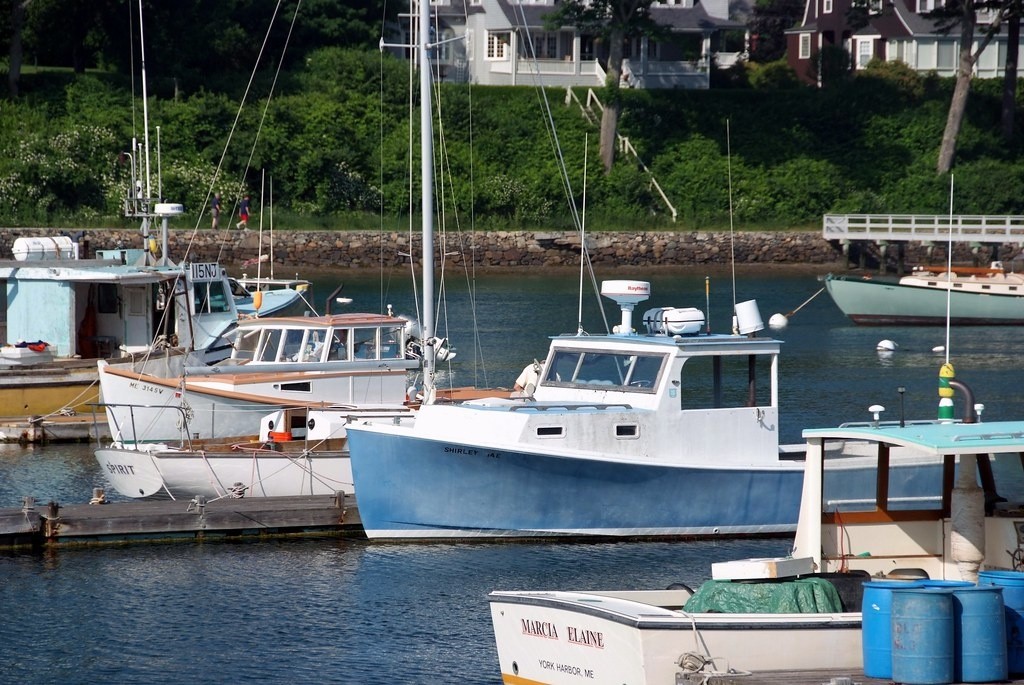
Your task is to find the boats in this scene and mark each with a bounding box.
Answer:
[817,263,1024,327]
[0,1,311,421]
[95,273,517,451]
[486,172,1024,684]
[345,127,998,549]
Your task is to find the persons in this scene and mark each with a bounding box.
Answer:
[211,193,221,229]
[236,195,251,230]
[513,358,561,391]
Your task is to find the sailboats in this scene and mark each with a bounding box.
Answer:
[85,0,652,502]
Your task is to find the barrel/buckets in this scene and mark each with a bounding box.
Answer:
[861,570,1024,685]
[735,300,765,335]
[274,432,292,442]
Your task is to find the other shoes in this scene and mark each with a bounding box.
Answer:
[236,223,241,229]
